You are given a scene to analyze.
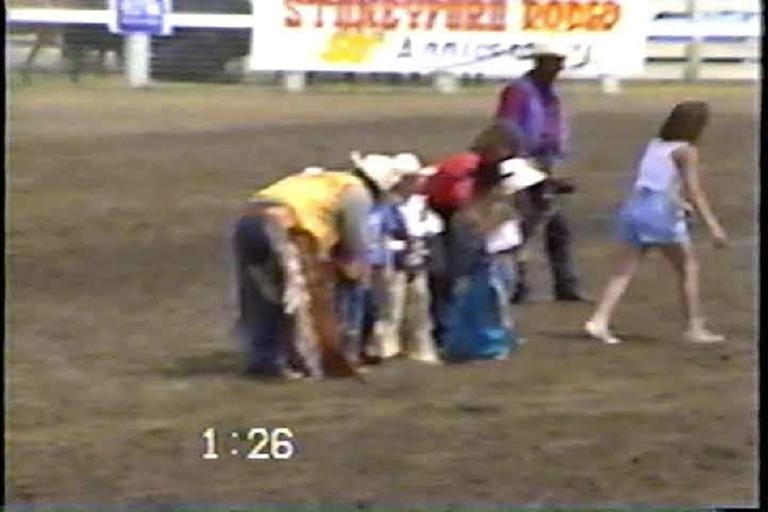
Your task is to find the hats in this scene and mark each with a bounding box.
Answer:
[347,146,549,242]
[518,37,577,60]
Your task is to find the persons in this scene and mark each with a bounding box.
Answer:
[496,46,594,303]
[230,122,548,384]
[583,101,729,346]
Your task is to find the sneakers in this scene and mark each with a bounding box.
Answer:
[681,327,726,344]
[584,316,622,344]
[557,281,595,302]
